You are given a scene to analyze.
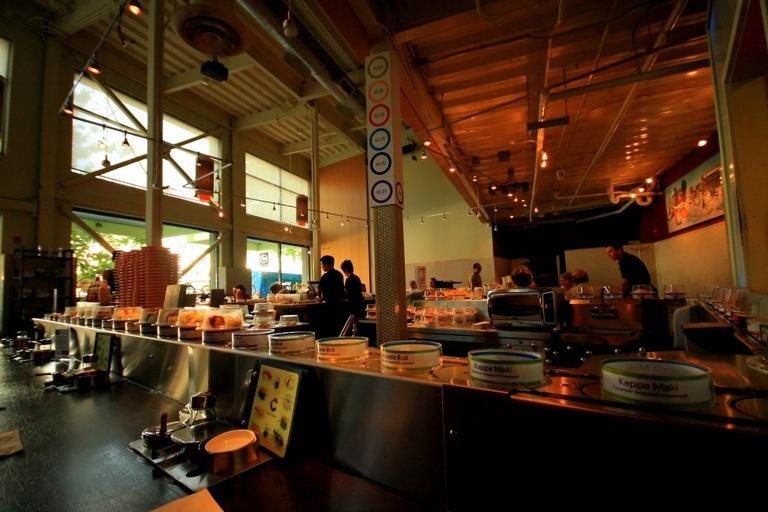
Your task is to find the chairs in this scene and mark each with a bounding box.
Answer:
[91,331,114,375]
[240,357,304,470]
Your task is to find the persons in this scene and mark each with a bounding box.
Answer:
[233,255,366,337]
[410,239,658,299]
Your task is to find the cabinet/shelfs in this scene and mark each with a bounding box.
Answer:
[9,245,73,336]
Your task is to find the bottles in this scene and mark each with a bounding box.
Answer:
[190,393,217,426]
[16,331,29,340]
[39,338,52,351]
[81,353,99,371]
